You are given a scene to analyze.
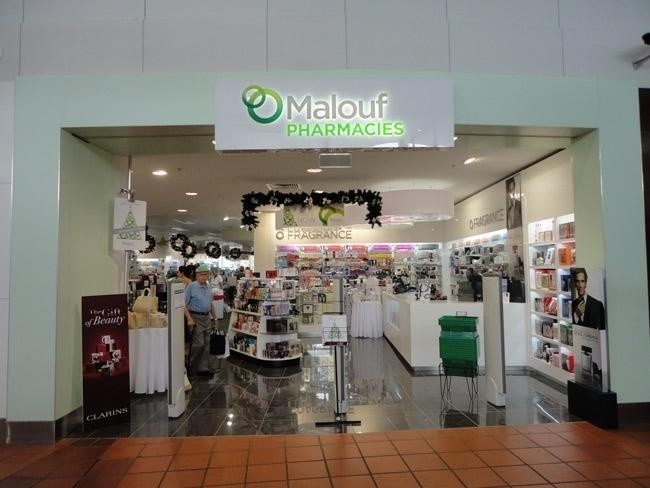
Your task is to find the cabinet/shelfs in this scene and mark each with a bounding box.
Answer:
[274,242,444,278]
[224,277,305,367]
[445,213,579,388]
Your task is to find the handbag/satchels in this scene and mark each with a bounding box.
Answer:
[210,328,230,360]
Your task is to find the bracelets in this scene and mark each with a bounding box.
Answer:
[213,317,218,321]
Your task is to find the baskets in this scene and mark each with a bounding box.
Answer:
[438,314,482,376]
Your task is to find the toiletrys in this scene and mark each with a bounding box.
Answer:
[230,281,302,359]
[533,250,574,373]
[580,344,593,372]
[91,334,123,378]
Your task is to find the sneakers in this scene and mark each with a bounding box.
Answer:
[198,370,215,378]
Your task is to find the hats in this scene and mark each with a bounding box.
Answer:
[195,265,210,272]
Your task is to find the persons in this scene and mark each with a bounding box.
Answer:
[244,266,252,278]
[571,268,606,330]
[234,266,245,279]
[185,266,218,375]
[177,265,192,290]
[209,267,225,320]
